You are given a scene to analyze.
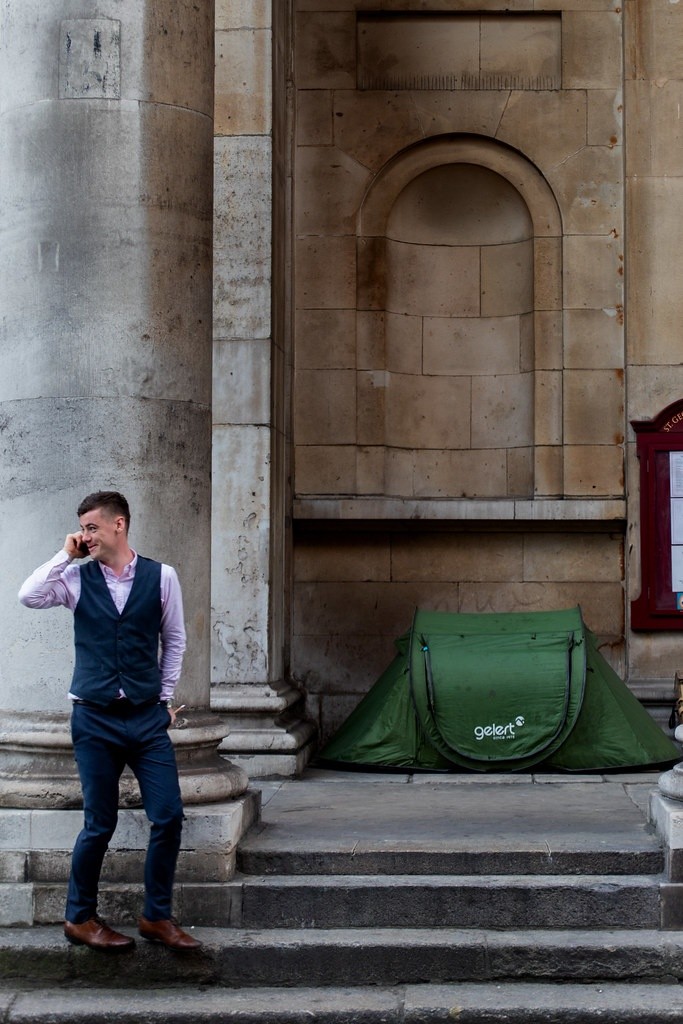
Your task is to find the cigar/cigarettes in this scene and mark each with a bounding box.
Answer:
[174,705,186,713]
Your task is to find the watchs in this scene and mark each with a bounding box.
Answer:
[159,699,172,708]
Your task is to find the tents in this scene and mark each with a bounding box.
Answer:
[310,603,683,772]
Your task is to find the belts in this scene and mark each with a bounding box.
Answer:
[74,696,160,707]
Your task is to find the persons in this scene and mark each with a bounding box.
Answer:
[18,489,203,953]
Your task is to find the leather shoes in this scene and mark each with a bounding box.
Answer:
[64,918,134,951]
[137,916,203,949]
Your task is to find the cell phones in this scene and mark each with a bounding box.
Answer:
[78,542,91,556]
